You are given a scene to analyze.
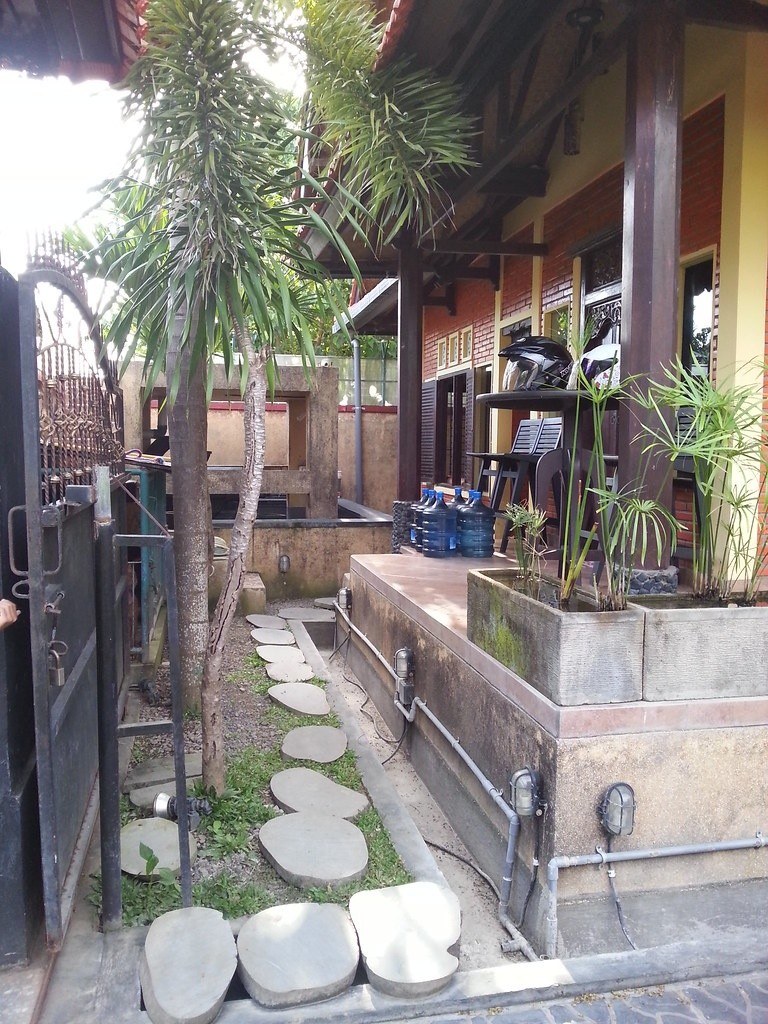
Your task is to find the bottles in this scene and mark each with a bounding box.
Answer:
[458,490,477,512]
[459,492,495,558]
[415,491,436,552]
[423,492,458,559]
[410,489,429,548]
[448,488,466,508]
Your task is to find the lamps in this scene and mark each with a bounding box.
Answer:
[393,646,415,680]
[507,766,543,816]
[336,587,352,609]
[279,555,290,574]
[598,783,636,837]
[153,792,212,820]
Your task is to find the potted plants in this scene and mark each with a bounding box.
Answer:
[466,312,641,704]
[586,308,767,708]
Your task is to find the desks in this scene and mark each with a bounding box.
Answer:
[474,389,620,586]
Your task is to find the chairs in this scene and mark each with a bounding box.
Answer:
[466,402,706,566]
